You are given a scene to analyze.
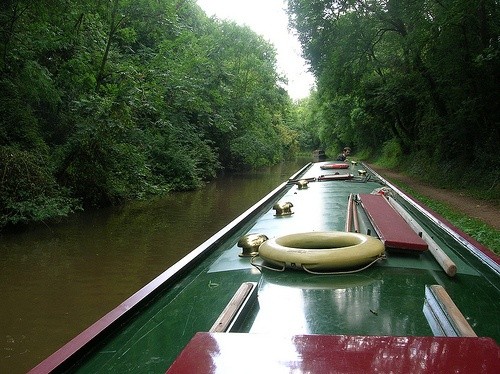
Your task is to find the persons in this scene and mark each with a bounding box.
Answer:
[336,147,351,162]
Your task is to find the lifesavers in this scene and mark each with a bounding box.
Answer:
[319,163,350,169]
[258,232,386,271]
